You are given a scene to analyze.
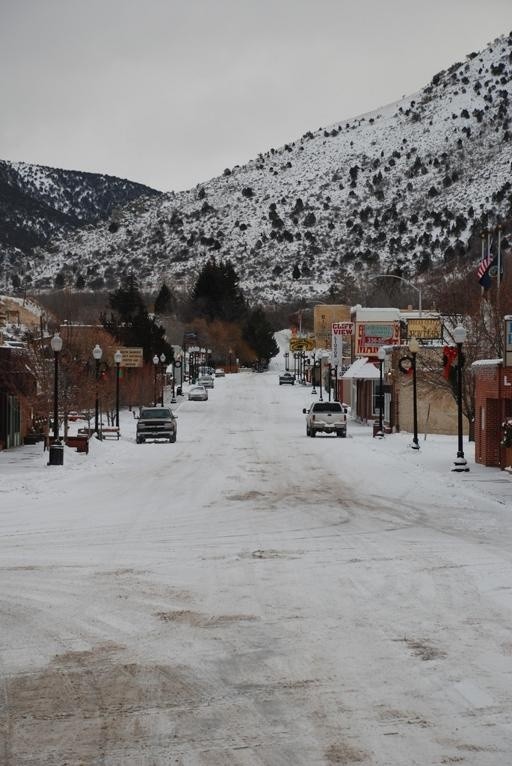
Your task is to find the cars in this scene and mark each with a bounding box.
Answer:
[215,368,225,377]
[279,373,294,385]
[188,377,214,401]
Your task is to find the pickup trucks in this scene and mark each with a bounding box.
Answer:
[136,407,178,444]
[303,402,347,438]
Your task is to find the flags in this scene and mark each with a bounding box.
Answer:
[477,230,503,291]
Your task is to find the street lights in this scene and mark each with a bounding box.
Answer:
[409,339,420,449]
[47,333,64,466]
[153,353,166,407]
[451,327,470,472]
[93,344,102,432]
[303,346,339,402]
[188,346,212,384]
[368,273,421,317]
[114,350,122,426]
[376,349,386,437]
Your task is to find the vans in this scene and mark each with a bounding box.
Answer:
[199,366,215,375]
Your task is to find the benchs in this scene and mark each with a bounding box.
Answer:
[44,434,89,453]
[78,427,121,440]
